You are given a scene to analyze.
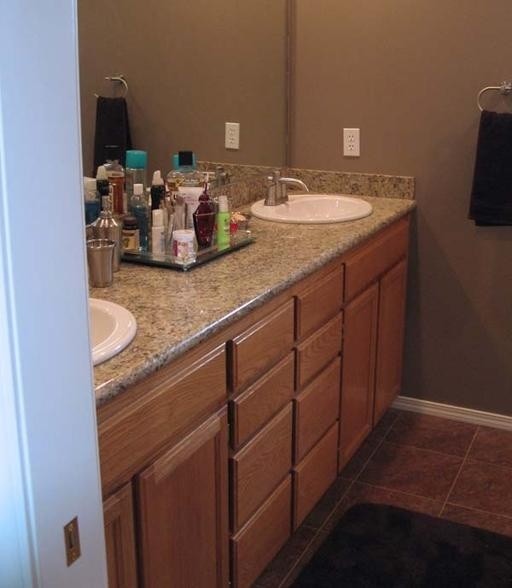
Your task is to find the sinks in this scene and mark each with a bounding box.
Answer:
[250,194,374,224]
[88,297,138,368]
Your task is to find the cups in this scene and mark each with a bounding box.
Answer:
[86,240,116,289]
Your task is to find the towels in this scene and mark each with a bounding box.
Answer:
[92,92,137,172]
[467,106,512,226]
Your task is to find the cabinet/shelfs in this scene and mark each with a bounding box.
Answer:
[229,271,344,578]
[95,343,226,585]
[338,225,412,492]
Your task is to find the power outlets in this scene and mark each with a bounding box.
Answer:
[341,126,361,157]
[223,120,241,150]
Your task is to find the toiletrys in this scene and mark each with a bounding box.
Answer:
[83,150,239,288]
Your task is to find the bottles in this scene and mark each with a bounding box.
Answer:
[90,197,121,273]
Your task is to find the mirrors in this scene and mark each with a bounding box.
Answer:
[78,0,294,214]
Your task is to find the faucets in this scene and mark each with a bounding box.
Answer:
[266,170,310,204]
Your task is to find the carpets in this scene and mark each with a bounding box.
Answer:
[298,503,509,586]
